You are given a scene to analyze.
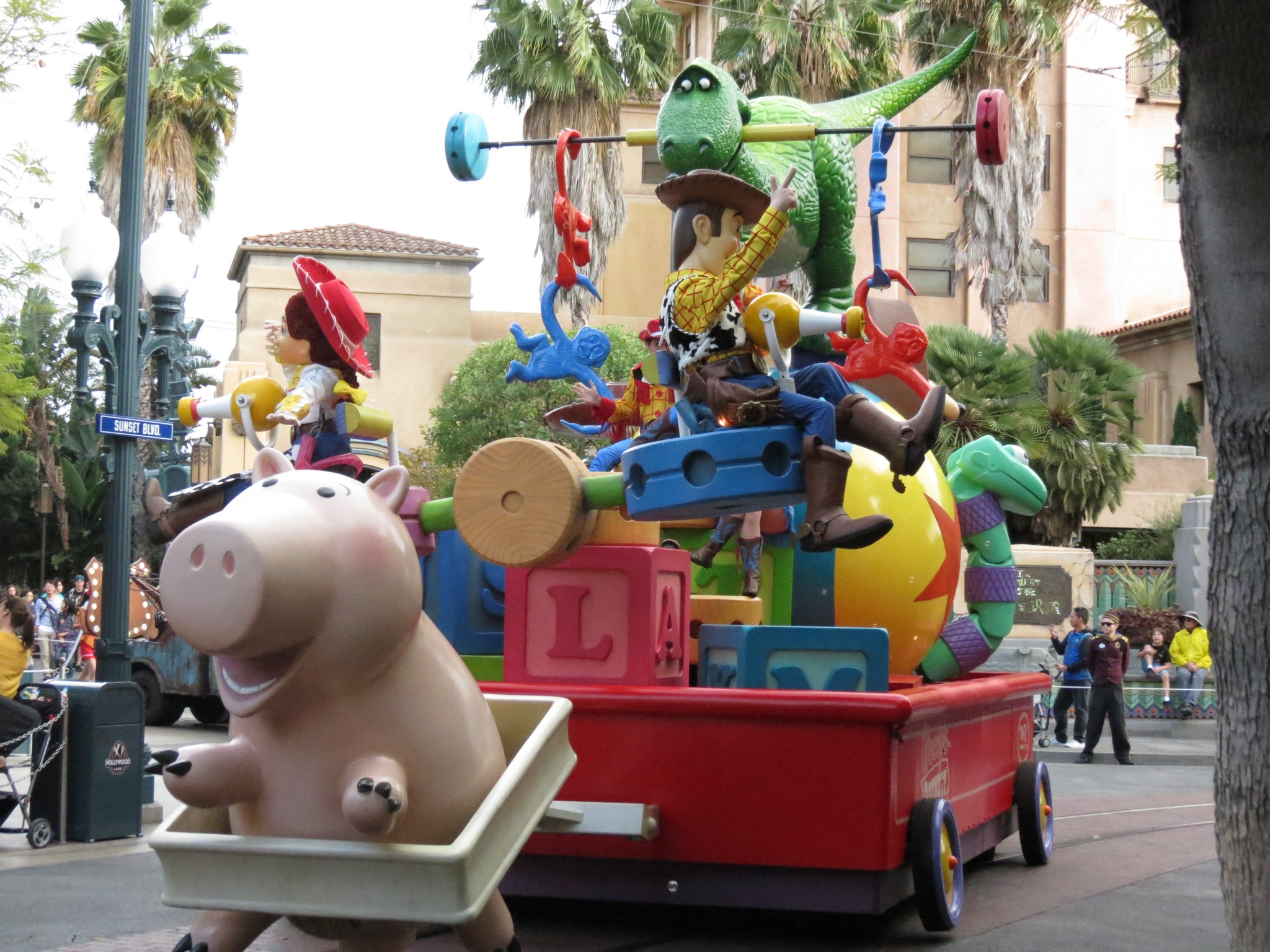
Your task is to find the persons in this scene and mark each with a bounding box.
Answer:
[1049,606,1095,748]
[53,599,76,679]
[572,319,671,471]
[0,596,35,700]
[65,575,89,672]
[141,256,376,545]
[84,581,90,588]
[1136,628,1172,705]
[1074,610,1134,766]
[6,585,18,596]
[656,168,945,554]
[35,580,64,681]
[690,504,796,597]
[20,589,36,667]
[71,589,96,682]
[1168,611,1213,716]
[41,577,65,600]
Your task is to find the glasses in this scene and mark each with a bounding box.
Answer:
[1182,617,1194,622]
[1100,622,1117,627]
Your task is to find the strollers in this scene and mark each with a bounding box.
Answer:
[1031,662,1063,748]
[0,683,58,848]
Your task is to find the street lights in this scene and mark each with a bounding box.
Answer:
[24,218,200,845]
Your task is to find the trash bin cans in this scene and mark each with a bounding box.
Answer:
[31,679,145,840]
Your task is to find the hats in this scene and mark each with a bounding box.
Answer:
[1177,611,1204,627]
[75,575,85,581]
[1103,611,1120,625]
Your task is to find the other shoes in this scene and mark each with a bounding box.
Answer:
[1180,704,1192,716]
[76,666,82,672]
[1163,697,1171,704]
[44,676,49,680]
[1120,759,1134,765]
[1147,669,1154,676]
[1049,738,1067,746]
[1075,755,1092,764]
[1065,740,1085,749]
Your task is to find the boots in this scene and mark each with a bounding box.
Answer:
[738,534,762,596]
[688,514,744,568]
